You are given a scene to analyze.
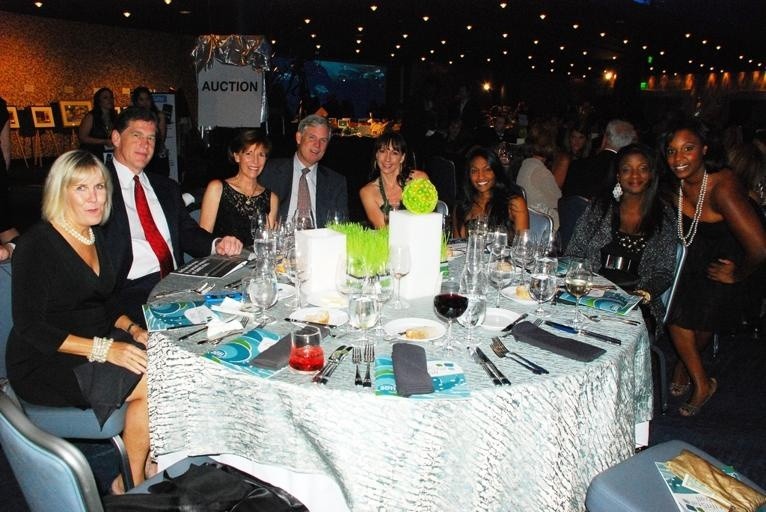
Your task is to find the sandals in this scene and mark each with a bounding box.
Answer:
[665,371,692,401]
[676,376,719,420]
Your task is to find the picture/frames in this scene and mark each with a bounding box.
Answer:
[30,105,56,128]
[6,105,20,131]
[57,100,92,128]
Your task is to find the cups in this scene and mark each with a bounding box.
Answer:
[248,275,277,328]
[527,261,558,318]
[604,253,633,272]
[278,214,294,234]
[459,233,488,295]
[363,270,396,342]
[347,292,382,348]
[485,222,508,254]
[325,205,345,228]
[288,325,326,376]
[563,258,594,327]
[292,207,316,230]
[540,229,562,259]
[286,247,314,313]
[250,212,270,238]
[510,228,539,288]
[455,291,486,344]
[253,228,276,260]
[432,270,470,360]
[384,243,413,311]
[334,252,370,334]
[486,244,518,308]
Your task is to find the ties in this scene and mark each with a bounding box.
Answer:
[297,167,312,229]
[132,173,175,279]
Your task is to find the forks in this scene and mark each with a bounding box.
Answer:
[156,282,209,291]
[153,283,219,299]
[195,315,251,344]
[489,343,542,375]
[492,337,549,375]
[351,346,363,385]
[501,318,544,338]
[362,343,376,387]
[209,321,267,343]
[581,314,640,327]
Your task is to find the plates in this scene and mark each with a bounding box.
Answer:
[383,317,447,343]
[276,282,298,301]
[289,306,350,329]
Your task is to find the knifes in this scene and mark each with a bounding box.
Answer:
[499,312,529,332]
[284,317,337,330]
[475,344,511,386]
[466,346,502,387]
[311,345,347,383]
[321,345,354,385]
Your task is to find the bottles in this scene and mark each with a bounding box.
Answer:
[461,224,488,328]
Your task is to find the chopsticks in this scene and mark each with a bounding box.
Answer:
[544,317,621,352]
[557,284,619,291]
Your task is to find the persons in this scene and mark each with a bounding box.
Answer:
[275,81,765,185]
[451,147,530,252]
[75,86,118,161]
[130,85,168,159]
[563,121,637,205]
[259,114,348,231]
[358,131,431,229]
[659,112,766,416]
[515,134,562,239]
[0,105,244,346]
[5,151,167,496]
[197,127,277,249]
[560,145,677,341]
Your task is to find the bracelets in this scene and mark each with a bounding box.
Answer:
[125,322,139,334]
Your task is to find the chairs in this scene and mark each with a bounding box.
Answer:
[437,200,448,224]
[586,440,766,512]
[183,208,202,264]
[1,378,310,512]
[423,158,456,216]
[0,260,133,491]
[527,206,553,250]
[558,194,592,255]
[650,235,688,416]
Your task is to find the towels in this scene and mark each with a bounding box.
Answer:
[391,341,435,400]
[512,318,606,371]
[249,321,330,372]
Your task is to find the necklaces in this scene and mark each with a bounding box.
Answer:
[54,214,96,246]
[678,172,708,250]
[234,173,258,206]
